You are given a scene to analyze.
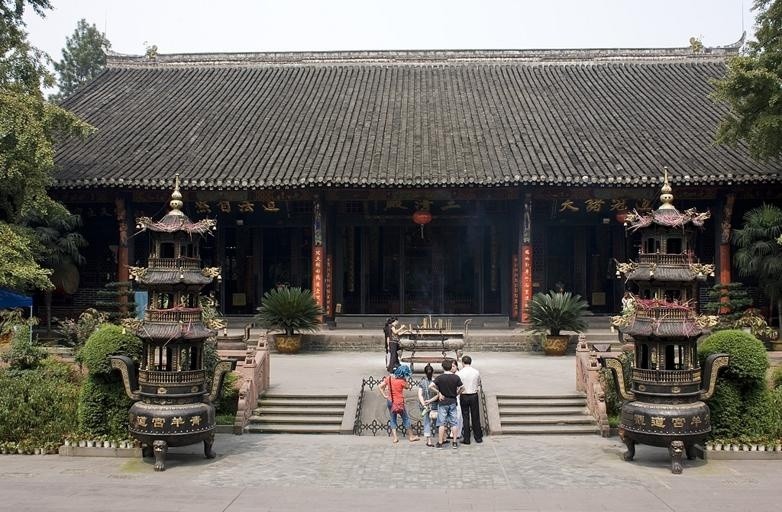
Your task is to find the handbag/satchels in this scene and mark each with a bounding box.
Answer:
[429,410,439,419]
[391,404,405,414]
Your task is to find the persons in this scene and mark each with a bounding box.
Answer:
[418,356,483,449]
[384,315,408,373]
[378,363,420,443]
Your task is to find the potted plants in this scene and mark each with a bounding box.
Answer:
[253,284,327,355]
[517,289,596,355]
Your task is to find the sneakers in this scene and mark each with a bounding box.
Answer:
[392,436,458,450]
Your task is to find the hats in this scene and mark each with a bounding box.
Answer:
[393,365,411,378]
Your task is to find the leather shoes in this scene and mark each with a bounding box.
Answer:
[460,436,483,445]
